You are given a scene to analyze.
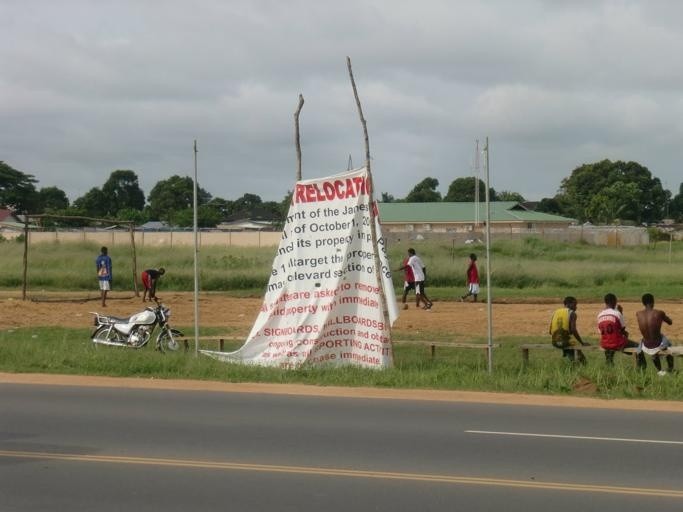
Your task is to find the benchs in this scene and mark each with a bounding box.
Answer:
[391,341,499,358]
[174,336,247,352]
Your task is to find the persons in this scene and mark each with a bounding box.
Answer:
[459,253,479,302]
[139,267,165,303]
[635,294,679,375]
[547,296,590,367]
[595,293,647,373]
[95,246,111,308]
[392,247,433,310]
[401,257,416,309]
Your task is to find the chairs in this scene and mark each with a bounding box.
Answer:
[518,342,683,370]
